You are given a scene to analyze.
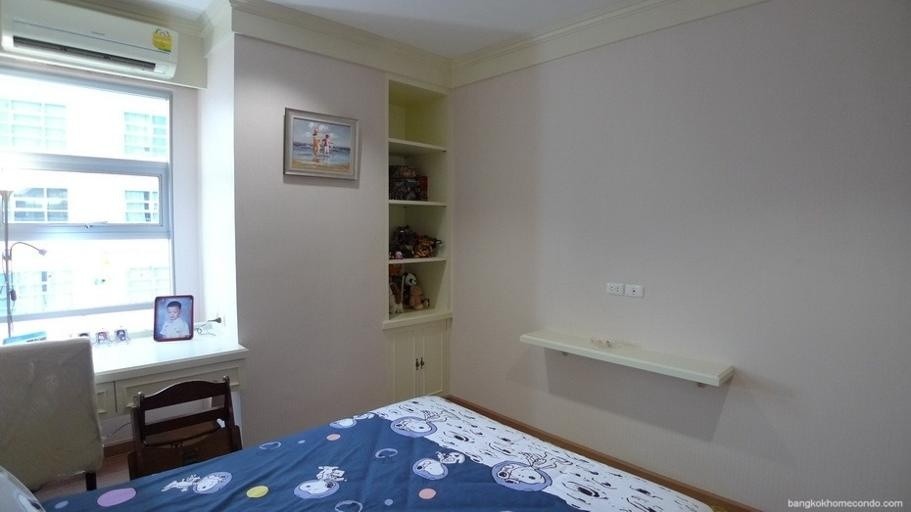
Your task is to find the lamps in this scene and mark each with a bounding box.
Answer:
[0,183,49,342]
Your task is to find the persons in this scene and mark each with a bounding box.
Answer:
[159,300,190,341]
[312,128,322,157]
[321,134,334,155]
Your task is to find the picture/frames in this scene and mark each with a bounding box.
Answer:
[154,295,193,340]
[283,106,361,181]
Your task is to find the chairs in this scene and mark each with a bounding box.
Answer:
[125,376,241,480]
[0,338,105,491]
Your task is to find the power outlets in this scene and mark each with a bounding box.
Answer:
[605,282,625,296]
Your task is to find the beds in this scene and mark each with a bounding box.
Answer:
[0,396,756,512]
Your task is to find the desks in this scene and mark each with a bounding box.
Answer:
[75,320,250,417]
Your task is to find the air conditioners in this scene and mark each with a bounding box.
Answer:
[0,0,180,80]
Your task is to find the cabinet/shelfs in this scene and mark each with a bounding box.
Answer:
[382,77,452,402]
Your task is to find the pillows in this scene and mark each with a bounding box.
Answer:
[0,465,46,512]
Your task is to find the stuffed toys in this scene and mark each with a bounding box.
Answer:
[389,222,443,260]
[389,270,431,314]
